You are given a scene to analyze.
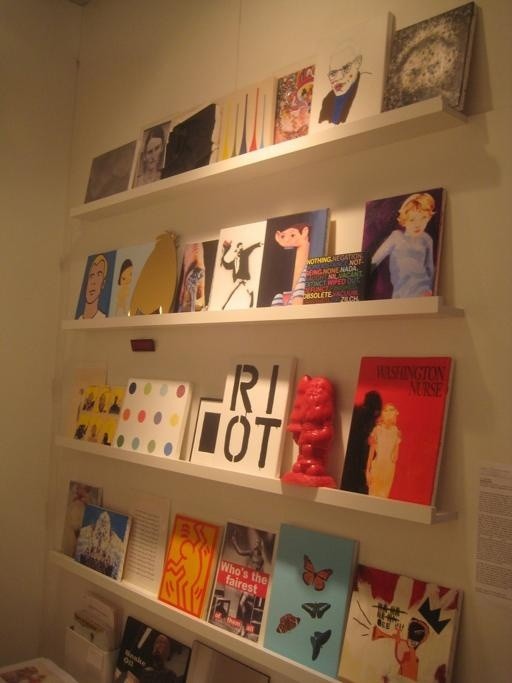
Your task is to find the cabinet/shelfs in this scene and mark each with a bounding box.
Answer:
[45,92,470,682]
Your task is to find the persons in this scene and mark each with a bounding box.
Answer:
[226,523,265,638]
[367,194,436,299]
[134,125,166,184]
[220,241,262,310]
